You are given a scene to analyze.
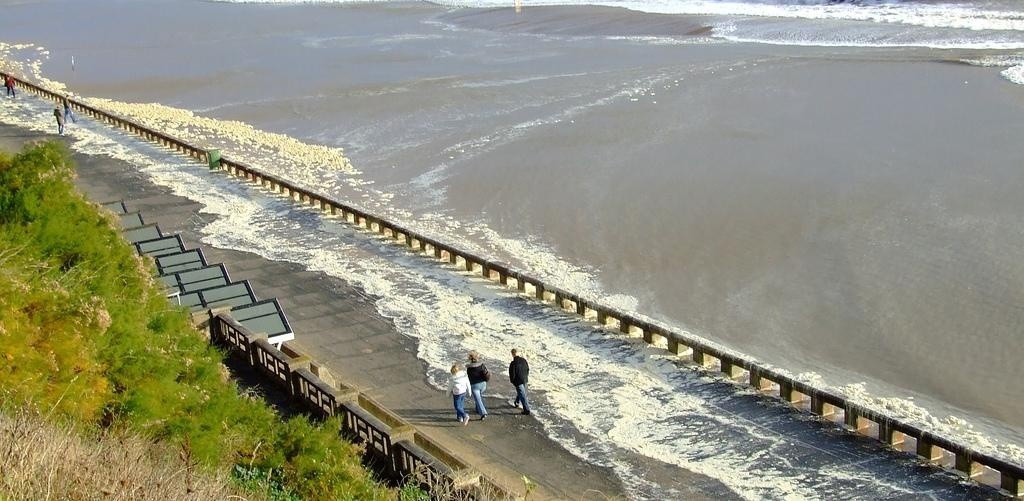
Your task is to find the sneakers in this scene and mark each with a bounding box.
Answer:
[514,400,530,415]
[480,414,488,421]
[456,414,470,426]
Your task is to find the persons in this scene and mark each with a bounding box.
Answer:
[508,349,530,417]
[466,353,490,420]
[446,363,472,426]
[53,106,66,137]
[3,72,16,99]
[62,94,77,124]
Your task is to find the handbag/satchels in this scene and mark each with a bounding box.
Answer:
[481,362,490,382]
